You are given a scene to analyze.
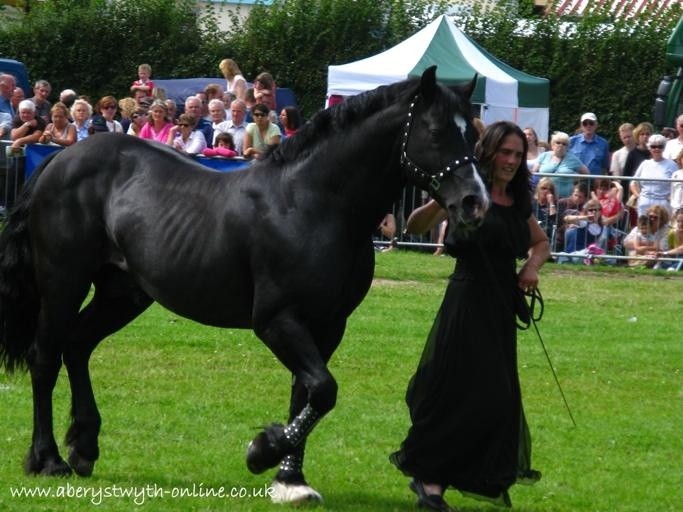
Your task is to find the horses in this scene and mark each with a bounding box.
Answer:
[0,65,490,506]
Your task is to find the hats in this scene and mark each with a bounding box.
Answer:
[580,112,597,123]
[139,97,153,106]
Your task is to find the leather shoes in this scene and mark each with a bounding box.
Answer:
[410,479,449,511]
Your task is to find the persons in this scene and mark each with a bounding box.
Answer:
[0,57,682,273]
[388,120,552,512]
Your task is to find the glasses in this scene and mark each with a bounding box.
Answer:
[650,145,663,149]
[253,112,266,117]
[588,208,601,212]
